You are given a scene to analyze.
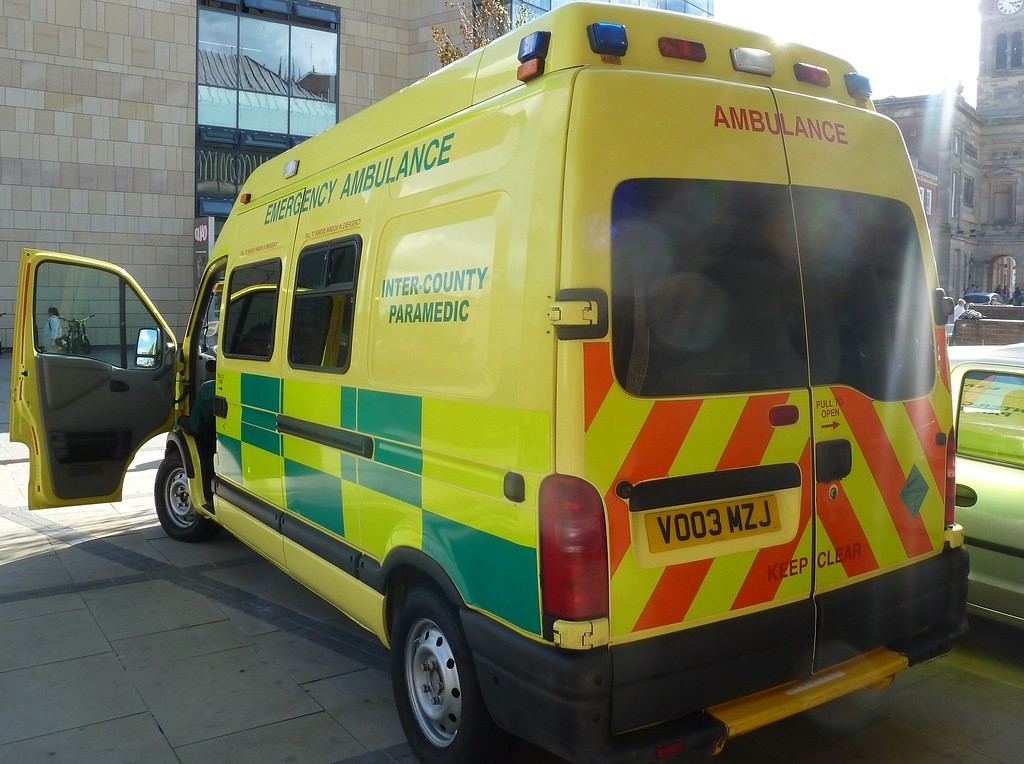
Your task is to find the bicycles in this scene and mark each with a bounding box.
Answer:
[57,313,95,355]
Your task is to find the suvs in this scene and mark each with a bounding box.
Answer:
[947,342,1024,626]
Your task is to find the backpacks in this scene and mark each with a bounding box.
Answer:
[958,310,982,319]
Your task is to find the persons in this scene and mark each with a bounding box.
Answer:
[949,299,967,346]
[43,307,64,353]
[177,379,215,438]
[964,283,1024,307]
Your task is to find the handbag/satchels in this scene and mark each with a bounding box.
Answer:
[55,335,70,351]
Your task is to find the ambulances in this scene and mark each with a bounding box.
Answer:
[13,3,971,764]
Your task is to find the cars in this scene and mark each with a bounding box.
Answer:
[963,292,1007,305]
